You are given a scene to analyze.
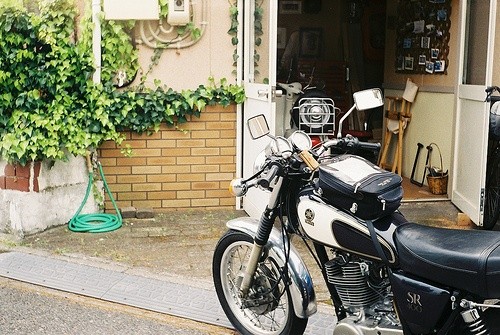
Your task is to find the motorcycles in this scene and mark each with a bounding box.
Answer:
[211,87,500,335]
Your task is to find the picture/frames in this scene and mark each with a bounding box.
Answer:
[277,0,322,57]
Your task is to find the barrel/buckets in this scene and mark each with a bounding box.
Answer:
[426,173,448,195]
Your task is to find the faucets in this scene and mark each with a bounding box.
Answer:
[96,161,101,165]
